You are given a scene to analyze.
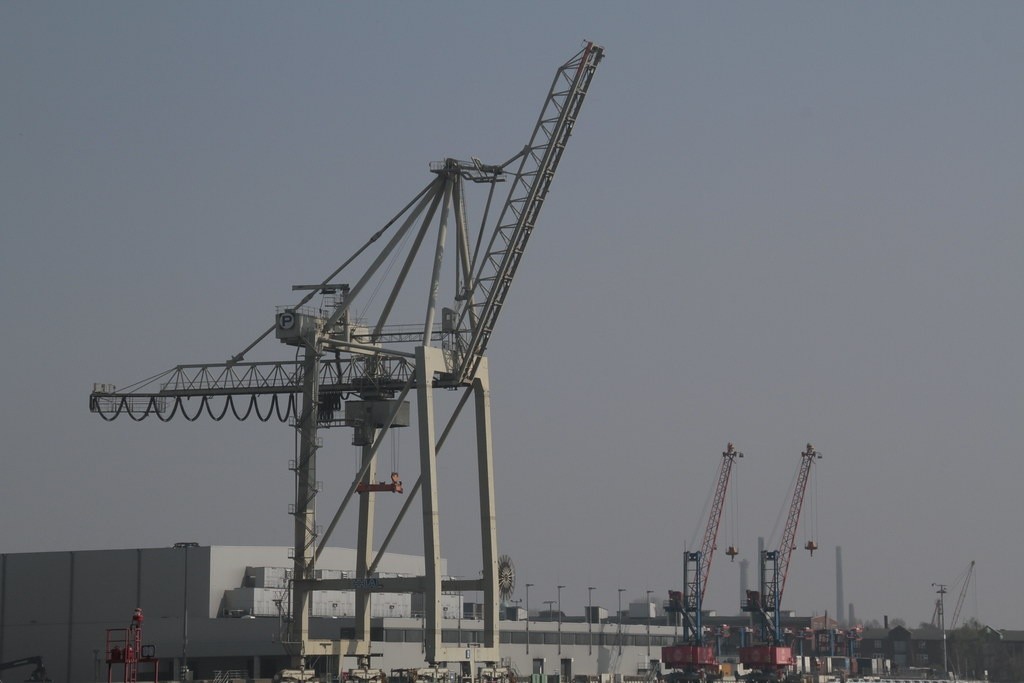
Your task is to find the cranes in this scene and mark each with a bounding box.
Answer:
[661,442,747,683]
[947,557,979,632]
[88,38,605,681]
[738,441,825,683]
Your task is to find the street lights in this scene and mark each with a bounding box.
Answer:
[169,540,201,682]
